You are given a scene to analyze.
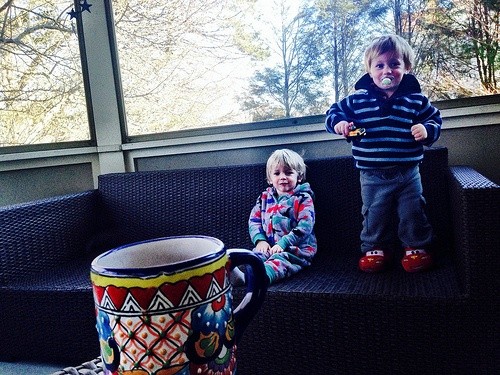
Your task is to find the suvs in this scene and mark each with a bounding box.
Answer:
[346,125,366,143]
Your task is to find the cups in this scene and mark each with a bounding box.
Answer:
[89,234,270,375]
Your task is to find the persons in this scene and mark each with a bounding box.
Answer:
[325,34,443,273]
[228,149,317,290]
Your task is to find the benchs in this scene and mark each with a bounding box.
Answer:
[0,147,500,375]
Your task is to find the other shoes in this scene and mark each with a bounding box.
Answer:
[359,248,387,271]
[402,246,430,271]
[228,266,245,286]
